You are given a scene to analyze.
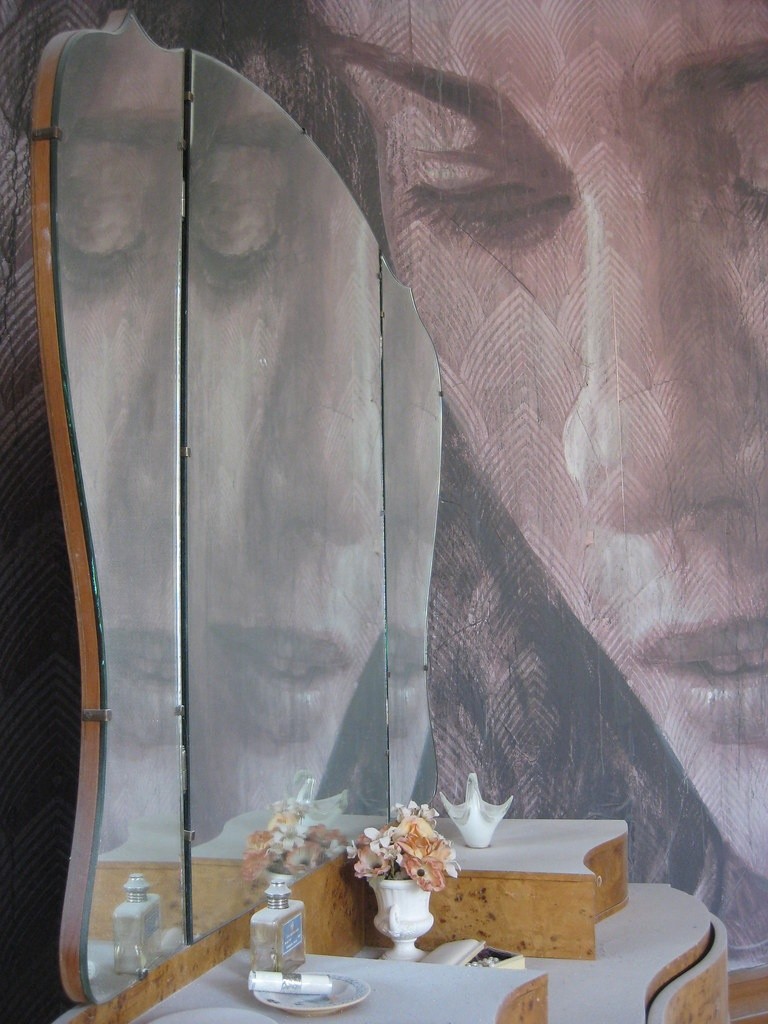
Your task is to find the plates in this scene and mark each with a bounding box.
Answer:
[147,1006,276,1024]
[253,971,372,1018]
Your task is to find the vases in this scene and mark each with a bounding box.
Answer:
[263,867,297,889]
[367,878,436,961]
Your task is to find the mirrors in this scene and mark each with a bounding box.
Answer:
[30,7,445,1005]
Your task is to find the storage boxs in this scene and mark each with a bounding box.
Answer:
[421,938,525,970]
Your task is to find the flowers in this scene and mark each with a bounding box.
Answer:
[242,799,345,878]
[347,802,462,893]
